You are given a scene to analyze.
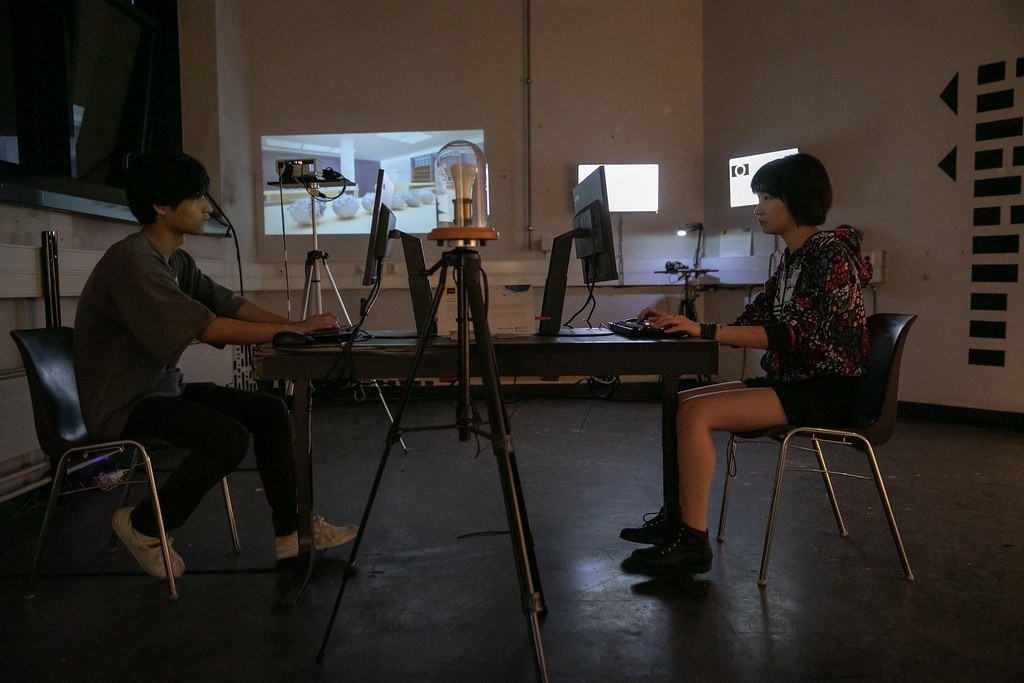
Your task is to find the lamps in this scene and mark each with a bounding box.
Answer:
[676,222,704,270]
[426,137,501,249]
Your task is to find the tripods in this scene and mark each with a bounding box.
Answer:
[287,183,409,455]
[312,249,551,683]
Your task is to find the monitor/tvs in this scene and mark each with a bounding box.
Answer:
[528,165,617,336]
[360,168,439,338]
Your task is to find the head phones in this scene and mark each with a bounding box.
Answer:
[664,260,689,270]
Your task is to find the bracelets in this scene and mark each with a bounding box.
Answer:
[699,322,717,340]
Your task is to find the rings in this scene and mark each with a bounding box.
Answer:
[673,313,676,318]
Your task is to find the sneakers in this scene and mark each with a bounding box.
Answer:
[274,516,356,559]
[620,505,714,575]
[112,506,185,579]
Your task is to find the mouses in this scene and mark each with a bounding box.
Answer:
[270,331,314,351]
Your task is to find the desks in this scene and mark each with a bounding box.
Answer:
[248,322,716,618]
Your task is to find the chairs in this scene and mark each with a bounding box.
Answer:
[715,311,917,585]
[10,328,243,604]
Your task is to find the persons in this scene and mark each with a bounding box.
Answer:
[621,154,875,577]
[74,149,359,581]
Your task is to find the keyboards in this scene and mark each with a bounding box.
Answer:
[312,324,354,343]
[603,320,686,339]
